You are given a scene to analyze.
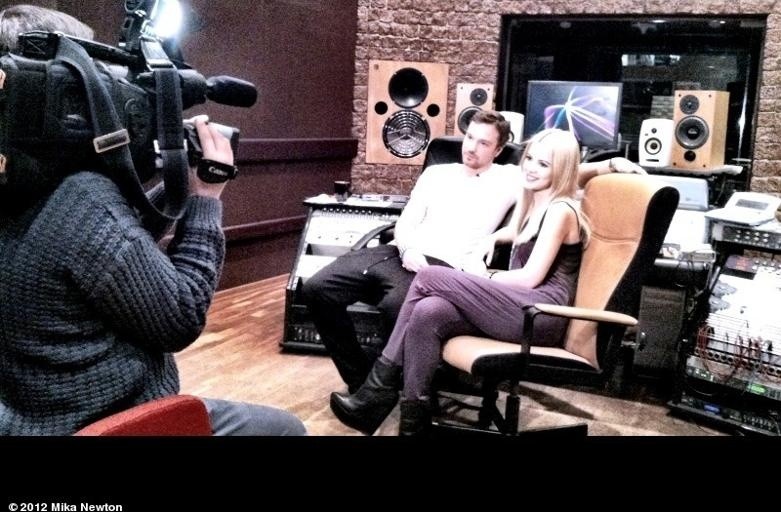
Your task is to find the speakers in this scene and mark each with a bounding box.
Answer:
[669,89,730,169]
[638,118,673,168]
[364,59,449,165]
[453,83,494,137]
[499,111,525,146]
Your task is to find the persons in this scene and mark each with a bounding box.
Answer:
[1,2,314,437]
[328,127,594,436]
[298,108,651,398]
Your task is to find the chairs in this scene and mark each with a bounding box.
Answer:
[72,394,213,436]
[439,173,680,435]
[350,136,523,332]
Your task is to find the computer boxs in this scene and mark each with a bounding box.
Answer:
[633,285,686,369]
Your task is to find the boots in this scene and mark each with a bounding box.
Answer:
[398,400,432,436]
[330,357,402,436]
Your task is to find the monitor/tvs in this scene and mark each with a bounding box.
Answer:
[522,81,624,151]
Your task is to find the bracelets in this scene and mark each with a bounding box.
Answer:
[607,160,620,174]
